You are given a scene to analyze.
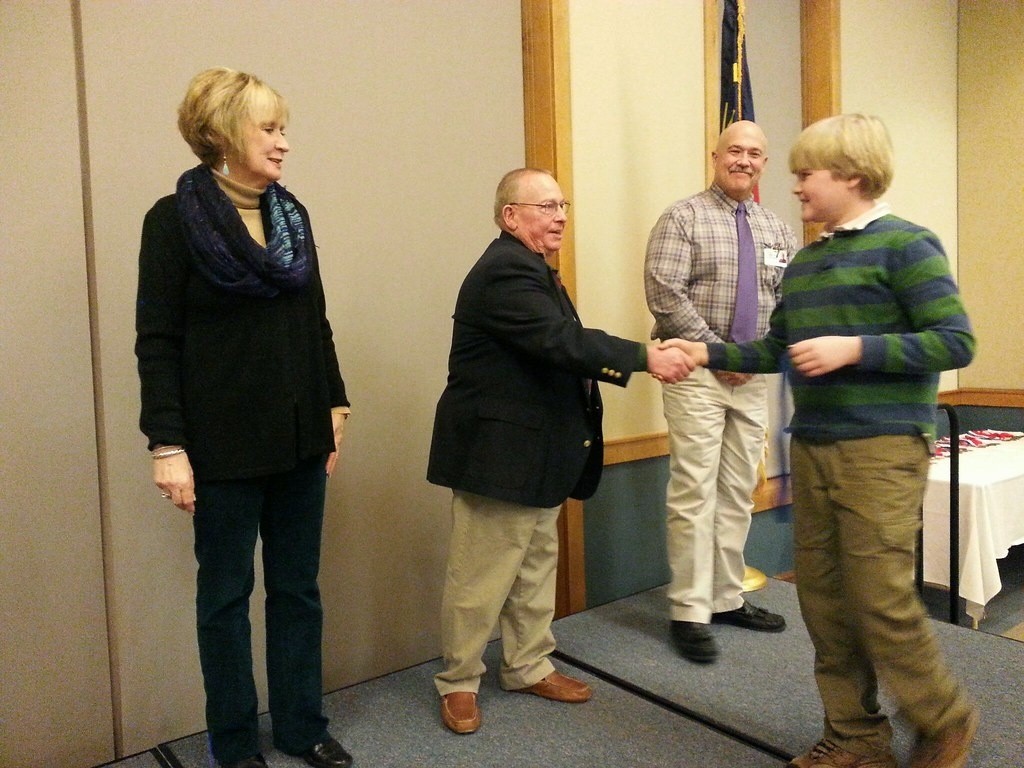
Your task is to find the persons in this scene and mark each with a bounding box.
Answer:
[653,112,980,768]
[644,120,799,661]
[133,68,354,768]
[426,170,694,733]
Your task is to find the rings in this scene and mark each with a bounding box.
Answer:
[162,492,172,499]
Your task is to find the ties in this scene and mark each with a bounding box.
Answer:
[730,202,758,343]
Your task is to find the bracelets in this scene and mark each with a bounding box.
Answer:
[151,449,185,459]
[150,446,174,455]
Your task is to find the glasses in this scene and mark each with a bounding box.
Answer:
[509,202,571,216]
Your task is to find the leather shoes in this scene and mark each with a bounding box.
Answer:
[273,734,353,768]
[711,600,786,633]
[512,670,592,702]
[670,620,719,660]
[218,752,268,768]
[440,692,482,734]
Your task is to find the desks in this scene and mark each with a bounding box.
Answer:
[913,428,1024,629]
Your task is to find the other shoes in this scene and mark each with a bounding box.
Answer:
[906,706,980,768]
[786,738,900,768]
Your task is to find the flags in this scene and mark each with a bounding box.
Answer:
[720,1,761,204]
